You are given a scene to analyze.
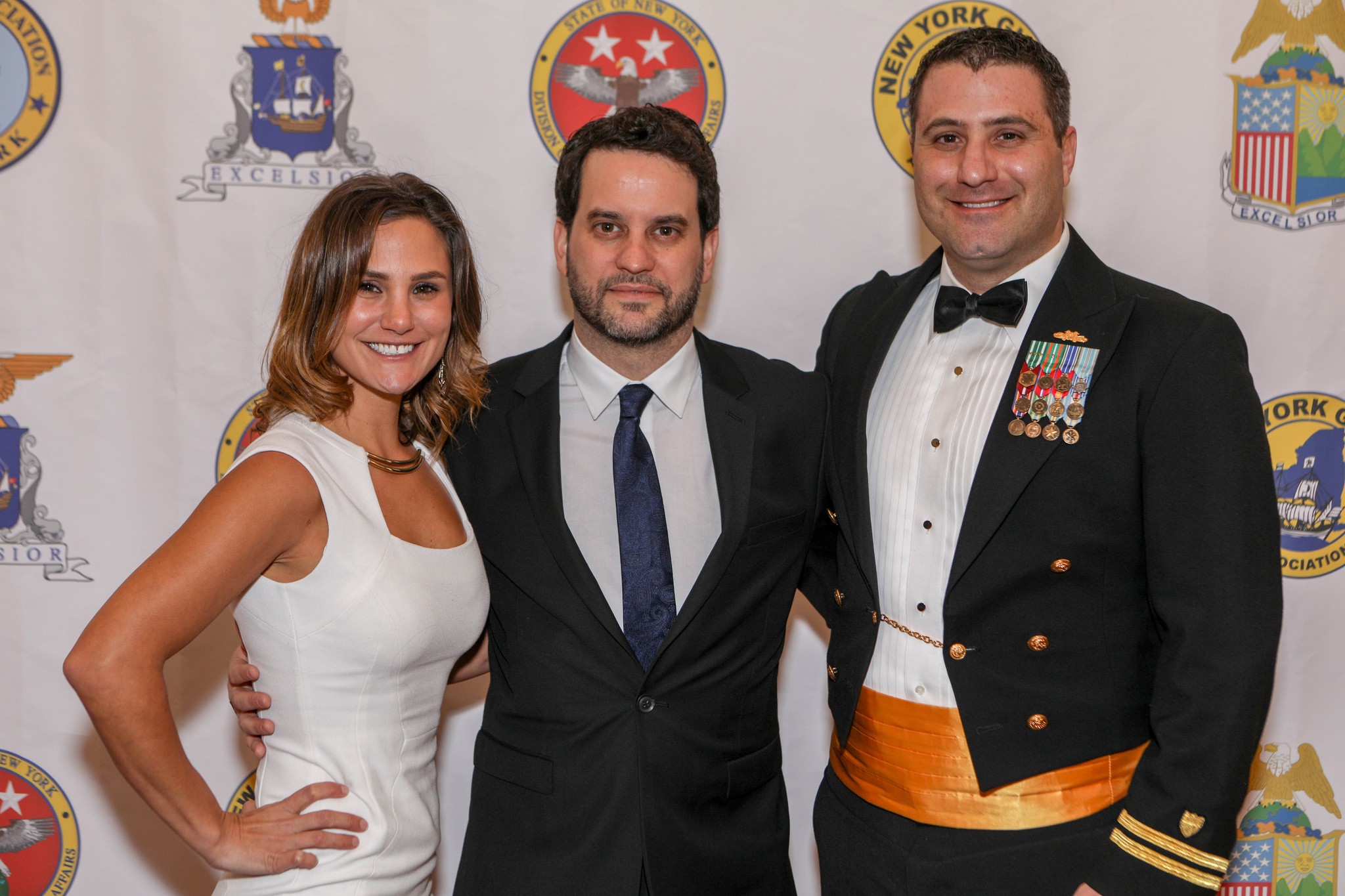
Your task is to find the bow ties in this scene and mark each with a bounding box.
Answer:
[933,279,1028,333]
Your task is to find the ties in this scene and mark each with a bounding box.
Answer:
[612,383,677,668]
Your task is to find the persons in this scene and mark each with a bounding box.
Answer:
[223,106,818,894]
[61,171,494,896]
[811,24,1283,896]
